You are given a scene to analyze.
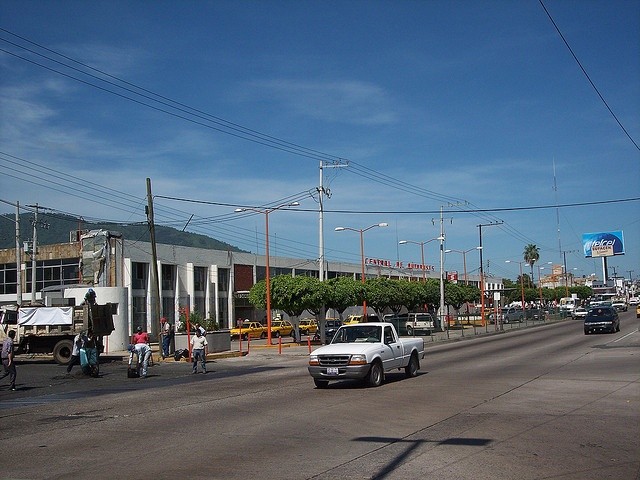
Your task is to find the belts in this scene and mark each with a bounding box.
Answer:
[194,349,204,350]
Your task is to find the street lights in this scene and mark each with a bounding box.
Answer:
[399,237,444,312]
[524,261,552,308]
[505,259,535,320]
[235,201,300,343]
[445,247,483,325]
[334,223,388,322]
[561,265,577,297]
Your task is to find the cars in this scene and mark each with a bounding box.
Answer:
[612,300,627,312]
[571,308,588,320]
[263,321,295,338]
[230,322,265,341]
[382,314,399,322]
[489,308,523,323]
[629,300,637,306]
[584,307,620,334]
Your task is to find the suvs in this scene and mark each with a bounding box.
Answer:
[392,313,435,336]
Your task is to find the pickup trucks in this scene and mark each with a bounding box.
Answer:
[299,319,317,335]
[308,322,425,387]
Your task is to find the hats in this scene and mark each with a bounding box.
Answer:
[160,318,166,324]
[127,344,135,353]
[137,327,142,331]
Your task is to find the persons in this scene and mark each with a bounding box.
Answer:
[262,316,267,338]
[189,329,209,374]
[188,320,207,337]
[244,318,250,322]
[126,342,152,379]
[236,317,244,328]
[158,318,171,358]
[80,288,97,305]
[0,330,17,391]
[66,330,92,373]
[134,325,149,344]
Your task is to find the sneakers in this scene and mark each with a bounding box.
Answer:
[192,370,197,374]
[203,369,206,374]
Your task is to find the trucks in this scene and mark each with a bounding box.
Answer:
[560,298,580,317]
[0,304,115,365]
